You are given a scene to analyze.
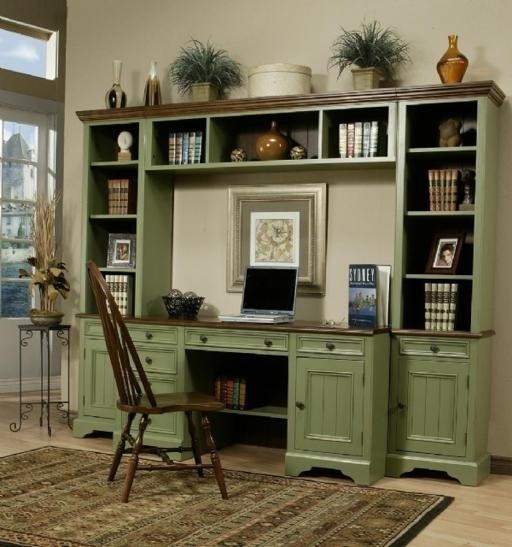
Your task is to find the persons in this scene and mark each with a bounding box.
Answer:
[438,244,454,266]
[119,245,128,259]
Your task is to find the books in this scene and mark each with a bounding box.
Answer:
[424,282,459,332]
[105,274,133,319]
[208,373,247,410]
[337,120,380,158]
[106,176,136,214]
[427,169,460,212]
[167,130,202,164]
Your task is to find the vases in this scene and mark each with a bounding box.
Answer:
[144,61,162,105]
[105,60,126,109]
[256,121,287,160]
[437,35,468,84]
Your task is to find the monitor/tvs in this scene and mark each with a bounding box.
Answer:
[217,264,300,325]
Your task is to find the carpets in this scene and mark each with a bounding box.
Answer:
[0,444,455,547]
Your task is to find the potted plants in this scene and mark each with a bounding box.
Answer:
[326,16,415,90]
[169,34,245,103]
[19,256,71,326]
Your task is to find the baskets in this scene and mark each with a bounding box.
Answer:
[161,295,206,320]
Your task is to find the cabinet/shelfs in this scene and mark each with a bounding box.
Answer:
[144,88,398,175]
[73,108,173,320]
[390,79,506,333]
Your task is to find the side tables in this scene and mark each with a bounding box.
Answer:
[9,325,73,436]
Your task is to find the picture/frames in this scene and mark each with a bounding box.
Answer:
[223,182,328,297]
[425,232,466,274]
[105,232,136,269]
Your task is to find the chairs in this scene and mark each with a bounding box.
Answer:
[85,258,230,503]
